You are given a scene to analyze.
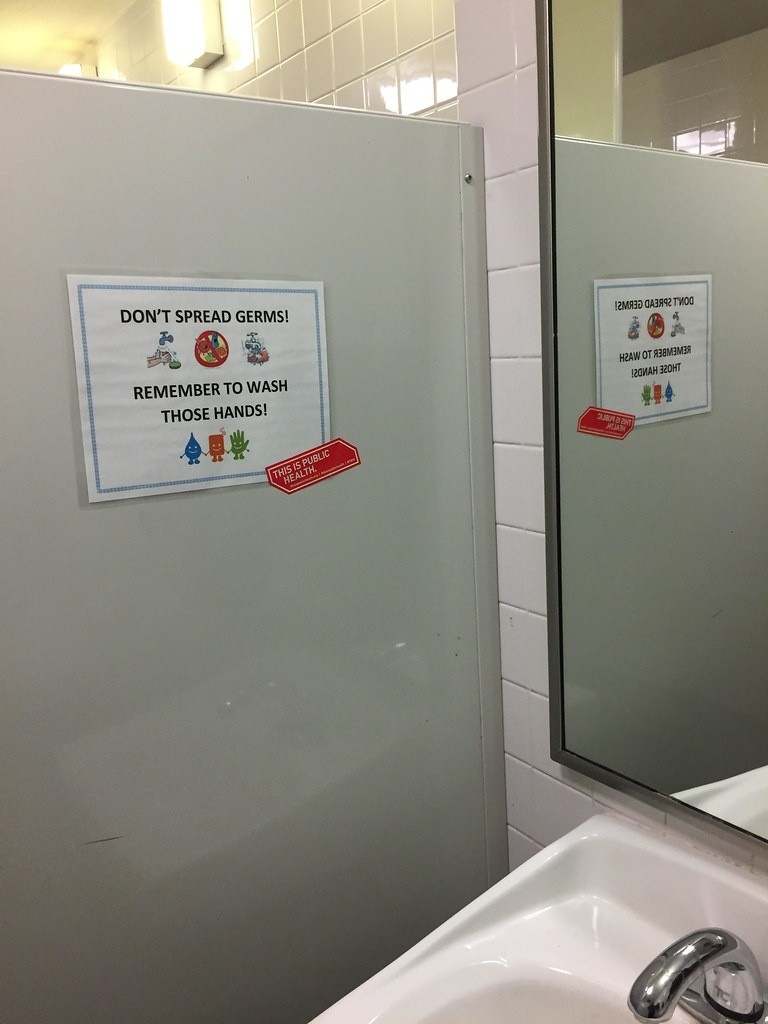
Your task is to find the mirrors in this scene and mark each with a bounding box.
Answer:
[531,0,768,847]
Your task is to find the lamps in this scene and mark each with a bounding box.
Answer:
[159,0,225,71]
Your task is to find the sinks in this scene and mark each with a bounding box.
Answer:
[411,956,690,1024]
[742,802,768,840]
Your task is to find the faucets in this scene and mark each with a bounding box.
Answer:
[626,924,768,1024]
